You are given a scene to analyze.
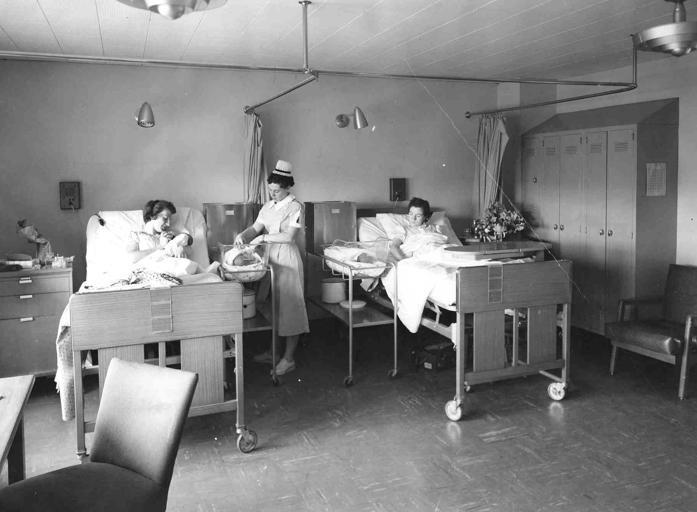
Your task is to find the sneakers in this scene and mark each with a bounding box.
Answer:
[254,352,280,364]
[270,358,296,376]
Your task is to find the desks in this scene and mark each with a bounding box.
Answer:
[442,240,552,262]
[0,375,36,485]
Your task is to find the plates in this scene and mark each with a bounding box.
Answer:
[340,300,366,308]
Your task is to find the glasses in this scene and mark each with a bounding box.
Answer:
[161,274,183,285]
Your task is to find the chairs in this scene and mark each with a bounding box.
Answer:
[0,356,199,512]
[604,263,697,401]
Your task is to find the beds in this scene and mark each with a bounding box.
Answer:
[355,207,574,422]
[55,206,258,463]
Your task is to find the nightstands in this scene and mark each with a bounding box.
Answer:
[0,263,74,379]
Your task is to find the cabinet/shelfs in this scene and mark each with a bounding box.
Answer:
[520,97,680,338]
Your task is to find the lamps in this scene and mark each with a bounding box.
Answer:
[137,102,156,128]
[632,0,697,62]
[335,105,368,131]
[118,0,226,21]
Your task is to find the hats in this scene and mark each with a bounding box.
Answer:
[272,160,293,177]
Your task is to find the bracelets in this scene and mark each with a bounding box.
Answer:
[261,233,265,244]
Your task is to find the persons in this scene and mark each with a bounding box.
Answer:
[118,199,194,265]
[389,195,444,262]
[233,159,310,377]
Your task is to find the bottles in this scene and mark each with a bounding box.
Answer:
[471,219,478,238]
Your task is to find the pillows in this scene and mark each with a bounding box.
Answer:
[375,211,464,249]
